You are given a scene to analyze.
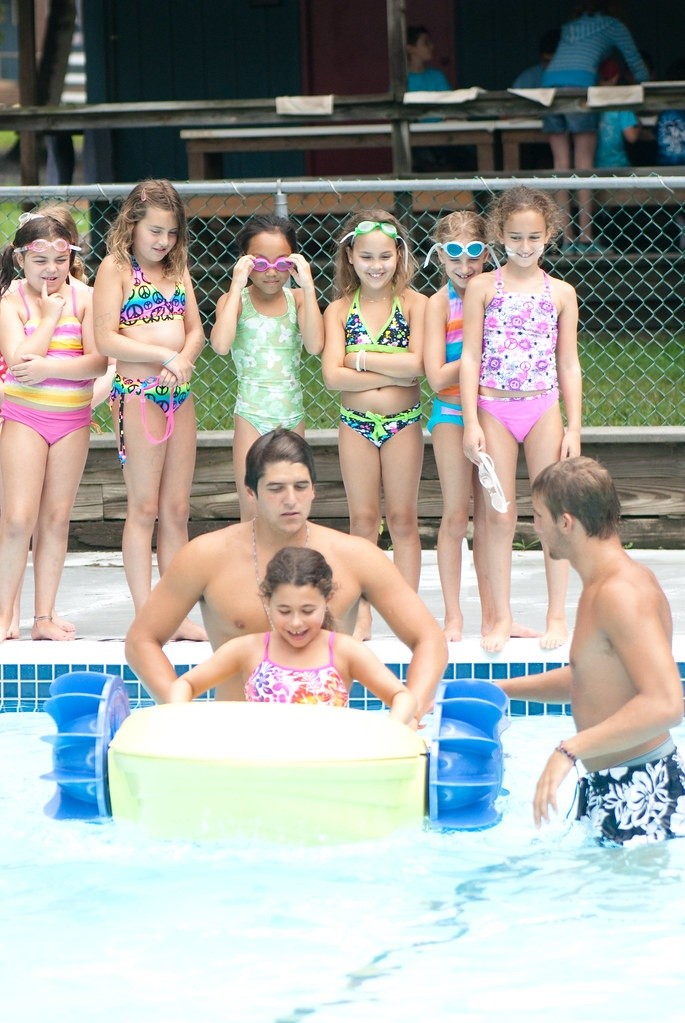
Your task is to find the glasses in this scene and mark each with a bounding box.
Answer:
[26,238,70,252]
[252,257,293,272]
[354,221,397,237]
[442,241,487,257]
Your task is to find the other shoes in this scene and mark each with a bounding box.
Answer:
[577,242,613,255]
[560,243,578,256]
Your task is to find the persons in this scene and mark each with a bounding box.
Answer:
[165,544,418,725]
[322,209,430,645]
[539,0,653,254]
[210,214,326,523]
[458,183,582,654]
[93,178,208,643]
[124,421,452,730]
[407,23,468,123]
[595,58,641,167]
[489,456,685,848]
[510,31,562,89]
[1,203,118,644]
[422,211,494,643]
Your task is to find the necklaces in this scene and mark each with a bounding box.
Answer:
[252,516,311,630]
[359,289,388,302]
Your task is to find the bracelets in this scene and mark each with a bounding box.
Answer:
[356,350,366,372]
[555,740,577,766]
[162,350,178,366]
